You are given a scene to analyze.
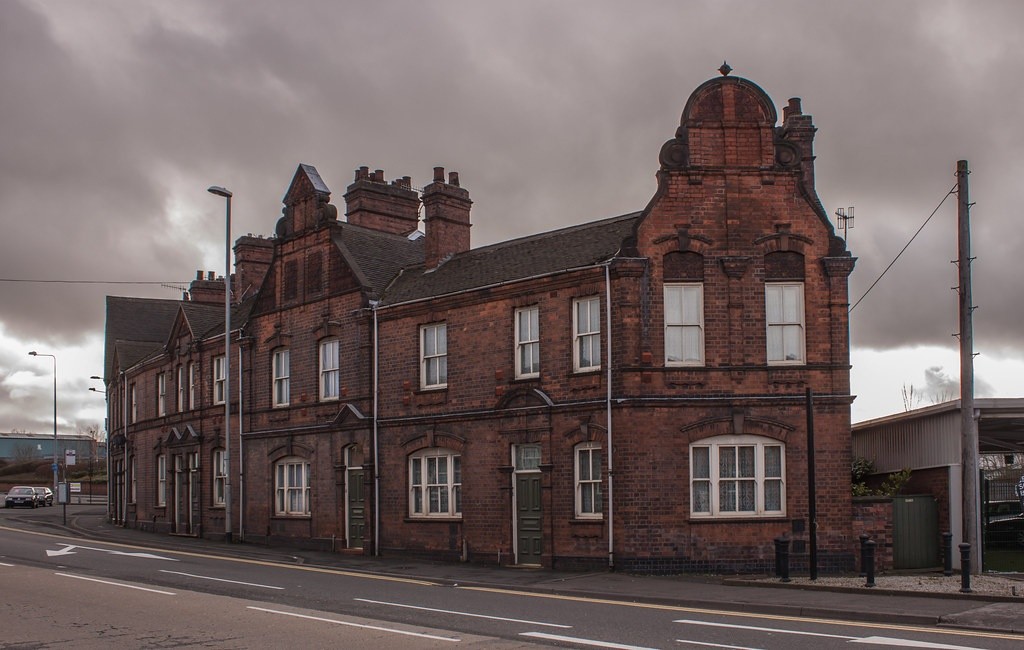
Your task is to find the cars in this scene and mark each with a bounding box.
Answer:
[33,487,54,506]
[4,486,36,508]
[982,500,1024,547]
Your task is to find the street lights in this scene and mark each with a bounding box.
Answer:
[29,351,58,504]
[207,185,233,542]
